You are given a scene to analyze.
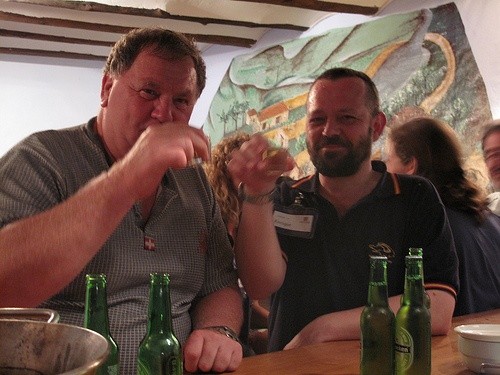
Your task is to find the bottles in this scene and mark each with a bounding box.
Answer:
[136,272,183,375]
[394,254,432,375]
[400,247,430,309]
[358,256,394,375]
[81,271,120,375]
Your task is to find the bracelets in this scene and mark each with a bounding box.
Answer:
[237,182,277,204]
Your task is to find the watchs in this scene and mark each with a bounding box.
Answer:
[199,326,243,345]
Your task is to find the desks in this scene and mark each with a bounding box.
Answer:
[221,309,500,375]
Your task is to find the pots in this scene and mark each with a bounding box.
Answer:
[0,307,111,375]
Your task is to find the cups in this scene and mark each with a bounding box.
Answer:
[453,324,500,375]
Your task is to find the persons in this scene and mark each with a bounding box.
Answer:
[480,119,500,216]
[386,117,500,316]
[227,68,460,353]
[0,25,255,375]
[201,131,271,346]
